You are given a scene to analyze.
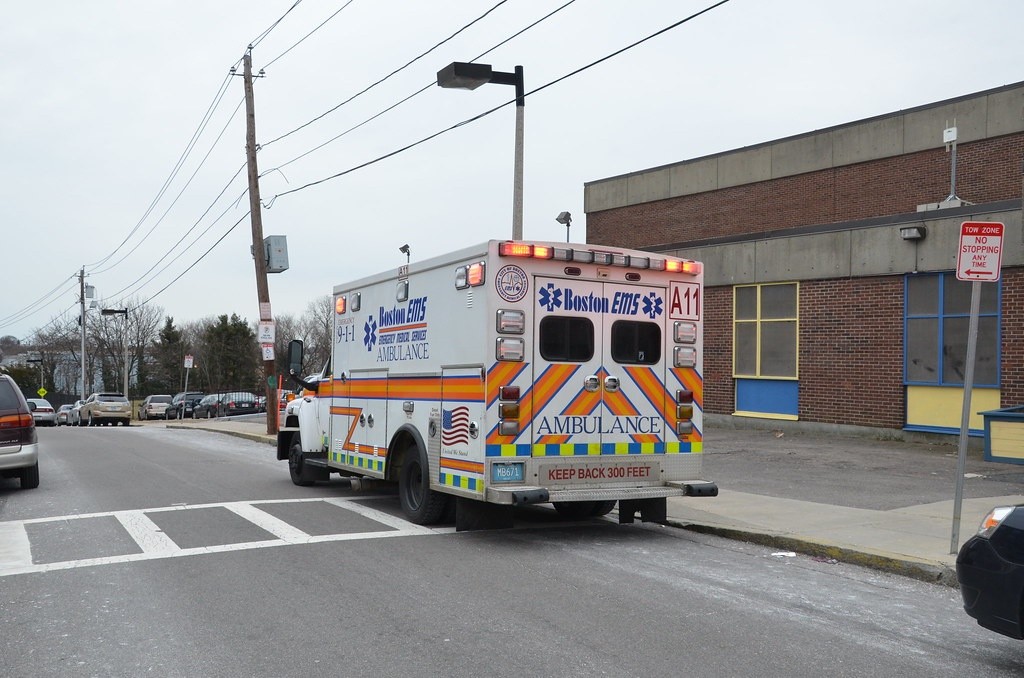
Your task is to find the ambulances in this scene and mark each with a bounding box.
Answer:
[272,238,718,533]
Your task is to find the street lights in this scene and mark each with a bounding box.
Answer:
[436,62,523,242]
[398,244,410,262]
[26,360,44,399]
[102,309,128,400]
[81,301,98,401]
[557,212,572,245]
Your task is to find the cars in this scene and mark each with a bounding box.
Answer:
[256,391,291,412]
[192,394,226,421]
[27,399,55,427]
[216,392,257,418]
[56,405,73,425]
[286,374,319,402]
[66,401,86,426]
[956,504,1024,640]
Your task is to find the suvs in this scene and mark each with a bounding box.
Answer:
[165,392,205,420]
[78,392,132,426]
[0,372,40,489]
[137,394,173,421]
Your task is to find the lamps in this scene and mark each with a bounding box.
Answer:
[899,226,926,241]
